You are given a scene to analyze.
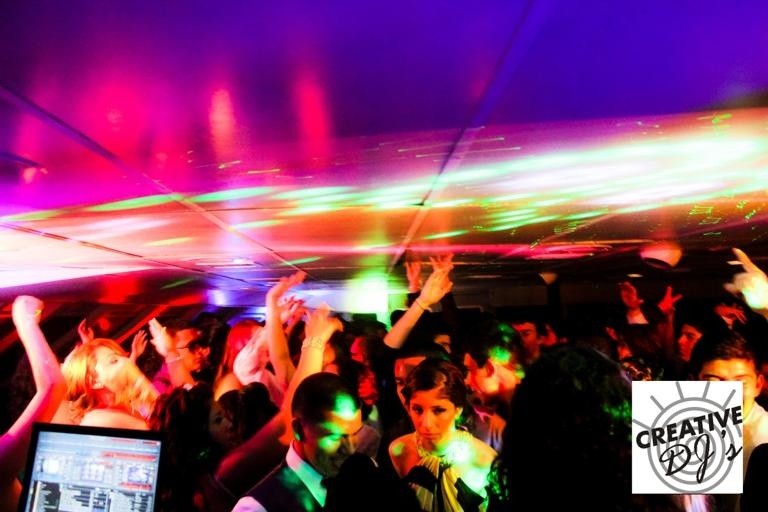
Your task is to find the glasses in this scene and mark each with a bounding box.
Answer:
[175,336,209,354]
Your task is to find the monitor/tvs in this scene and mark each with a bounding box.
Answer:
[17,420,168,512]
